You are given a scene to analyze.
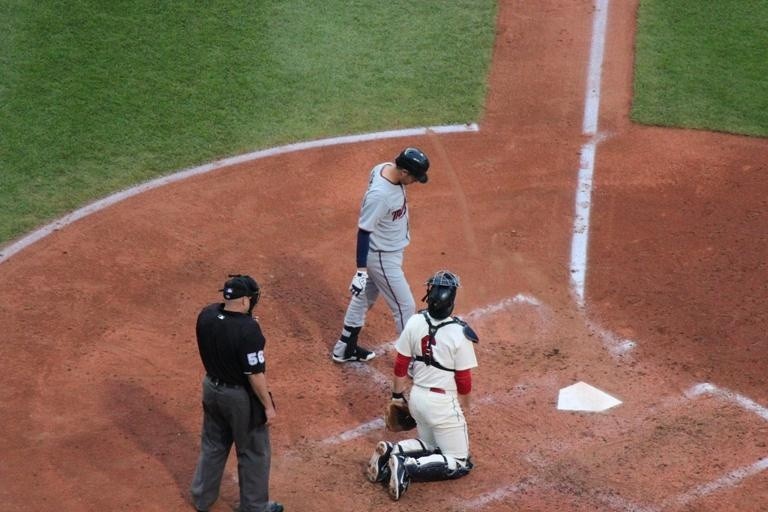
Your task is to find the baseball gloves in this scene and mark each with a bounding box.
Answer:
[386,404,416,432]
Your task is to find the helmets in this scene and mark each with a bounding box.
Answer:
[219,273,260,300]
[394,145,432,185]
[424,280,458,320]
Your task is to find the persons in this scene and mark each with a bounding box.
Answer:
[188,273,286,512]
[331,147,428,363]
[366,269,479,502]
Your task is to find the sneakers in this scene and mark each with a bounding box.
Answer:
[330,344,376,363]
[366,440,394,483]
[256,500,285,512]
[193,504,210,512]
[386,454,411,501]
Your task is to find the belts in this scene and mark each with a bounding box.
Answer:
[205,373,248,389]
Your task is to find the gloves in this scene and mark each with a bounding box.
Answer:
[347,269,369,298]
